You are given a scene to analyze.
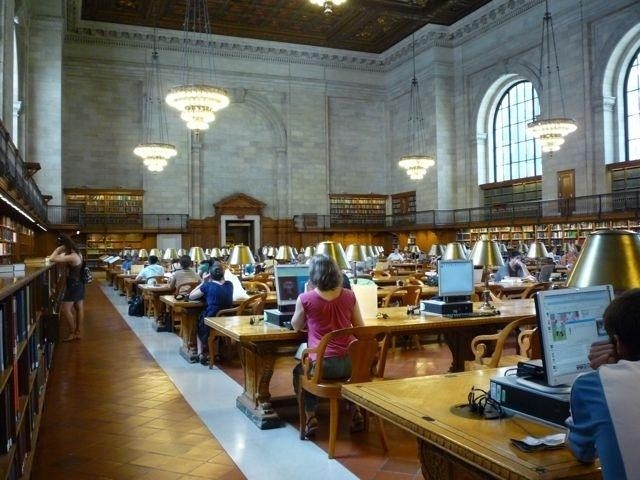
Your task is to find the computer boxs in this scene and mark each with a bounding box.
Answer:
[420,300,473,317]
[489,373,578,429]
[264,309,294,326]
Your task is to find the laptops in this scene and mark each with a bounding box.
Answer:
[532,265,555,282]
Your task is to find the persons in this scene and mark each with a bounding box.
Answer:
[386,248,416,263]
[50,234,85,342]
[491,247,580,288]
[122,253,250,364]
[252,251,307,276]
[291,253,366,437]
[563,288,640,480]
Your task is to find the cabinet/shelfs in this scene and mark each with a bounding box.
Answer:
[328,189,417,226]
[455,213,640,253]
[0,123,71,479]
[63,186,145,226]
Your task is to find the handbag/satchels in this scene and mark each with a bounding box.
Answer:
[242,264,261,280]
[129,296,144,317]
[83,267,93,283]
[428,276,438,286]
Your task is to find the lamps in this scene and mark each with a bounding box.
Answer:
[132,0,179,174]
[396,0,436,183]
[525,1,578,154]
[308,0,348,16]
[163,0,232,132]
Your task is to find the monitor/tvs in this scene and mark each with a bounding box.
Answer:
[274,264,315,306]
[438,260,475,296]
[533,284,615,387]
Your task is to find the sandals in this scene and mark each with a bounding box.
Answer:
[214,354,221,362]
[350,410,364,433]
[63,330,80,342]
[305,416,319,436]
[199,353,207,364]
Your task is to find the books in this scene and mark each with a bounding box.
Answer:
[328,194,418,226]
[602,220,640,229]
[459,220,602,250]
[67,193,141,224]
[0,210,67,479]
